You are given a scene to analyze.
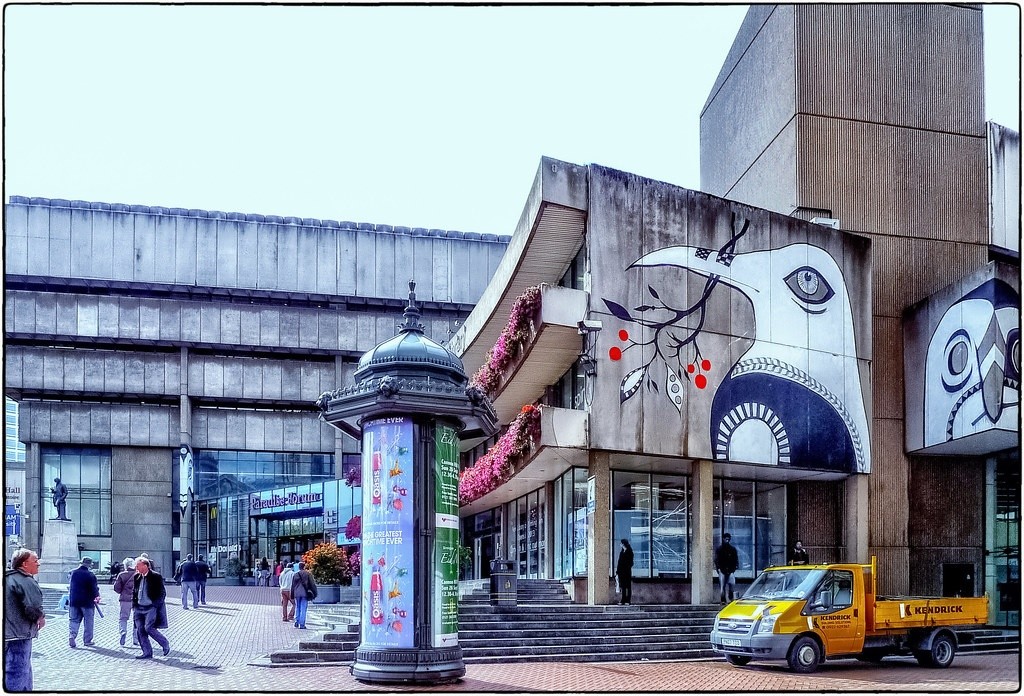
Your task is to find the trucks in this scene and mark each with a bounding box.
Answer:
[709,556,991,672]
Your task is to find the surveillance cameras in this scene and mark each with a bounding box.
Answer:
[167,493,171,497]
[576,320,602,331]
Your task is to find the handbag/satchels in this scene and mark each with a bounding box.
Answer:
[306,589,315,601]
[615,576,622,593]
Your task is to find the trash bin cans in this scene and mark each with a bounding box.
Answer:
[489,558,518,606]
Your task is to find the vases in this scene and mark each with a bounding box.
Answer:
[311,585,341,604]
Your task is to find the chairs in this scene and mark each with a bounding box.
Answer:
[835,579,852,604]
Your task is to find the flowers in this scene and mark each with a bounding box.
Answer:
[345,465,361,488]
[460,404,542,505]
[467,282,541,393]
[345,515,361,542]
[300,542,350,586]
[348,552,360,576]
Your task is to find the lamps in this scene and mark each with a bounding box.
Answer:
[439,320,462,345]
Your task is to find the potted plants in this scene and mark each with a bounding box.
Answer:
[223,557,244,586]
[458,545,473,580]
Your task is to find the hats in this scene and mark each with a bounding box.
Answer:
[80,558,94,566]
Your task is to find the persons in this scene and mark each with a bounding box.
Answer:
[194,554,212,605]
[273,559,299,622]
[259,556,270,586]
[6,548,46,691]
[615,538,634,606]
[290,562,314,629]
[792,540,809,565]
[179,554,198,609]
[108,553,169,659]
[715,532,738,605]
[68,557,101,647]
[51,478,68,518]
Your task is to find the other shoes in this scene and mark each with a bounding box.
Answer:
[163,649,169,656]
[294,622,298,627]
[134,642,138,645]
[136,653,152,659]
[84,642,95,646]
[300,626,306,629]
[69,637,76,647]
[120,633,125,645]
